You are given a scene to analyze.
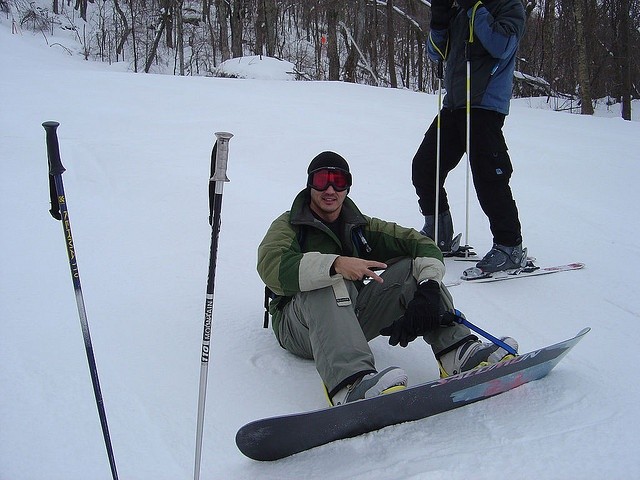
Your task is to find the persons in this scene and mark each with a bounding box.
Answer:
[256,150,517,407]
[411,0,529,273]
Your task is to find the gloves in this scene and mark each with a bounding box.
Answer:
[456,1,479,11]
[403,280,441,340]
[430,0,453,30]
[379,310,453,347]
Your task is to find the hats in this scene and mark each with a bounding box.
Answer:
[307,151,349,172]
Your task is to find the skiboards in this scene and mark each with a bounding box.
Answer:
[441,240,586,290]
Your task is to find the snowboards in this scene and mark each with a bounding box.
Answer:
[236,327,591,461]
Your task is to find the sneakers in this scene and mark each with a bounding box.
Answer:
[332,366,407,406]
[419,210,451,253]
[475,246,522,273]
[439,336,518,381]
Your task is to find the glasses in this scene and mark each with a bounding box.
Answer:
[310,172,350,191]
